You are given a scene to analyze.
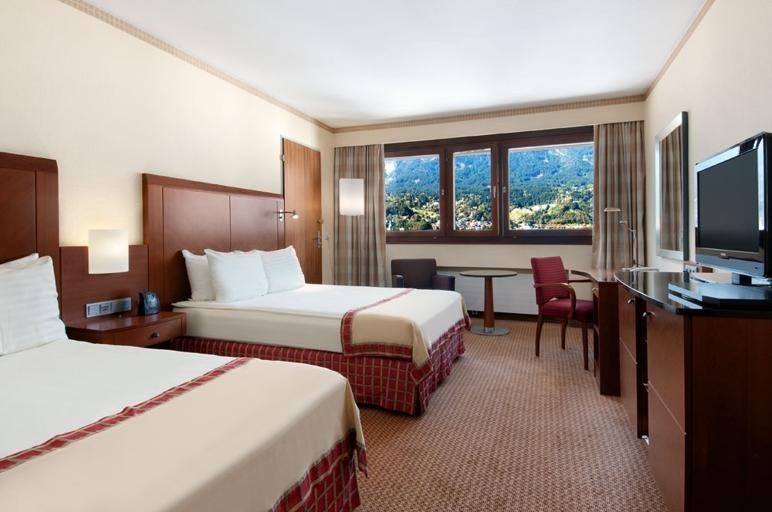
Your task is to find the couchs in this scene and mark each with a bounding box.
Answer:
[391,258,455,290]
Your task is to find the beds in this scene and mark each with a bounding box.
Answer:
[173,283,462,416]
[1,337,366,511]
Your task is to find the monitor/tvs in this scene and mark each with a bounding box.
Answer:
[668,131,772,306]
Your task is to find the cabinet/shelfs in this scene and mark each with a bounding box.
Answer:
[590,279,621,396]
[613,281,770,512]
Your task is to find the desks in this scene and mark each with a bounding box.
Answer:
[459,269,518,336]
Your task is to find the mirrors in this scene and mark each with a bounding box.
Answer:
[655,112,688,263]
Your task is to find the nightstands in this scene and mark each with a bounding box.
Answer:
[64,313,188,353]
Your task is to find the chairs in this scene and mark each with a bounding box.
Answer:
[531,255,593,371]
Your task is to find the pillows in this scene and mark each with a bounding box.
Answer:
[1,256,66,358]
[260,245,305,295]
[182,248,215,300]
[204,248,268,301]
